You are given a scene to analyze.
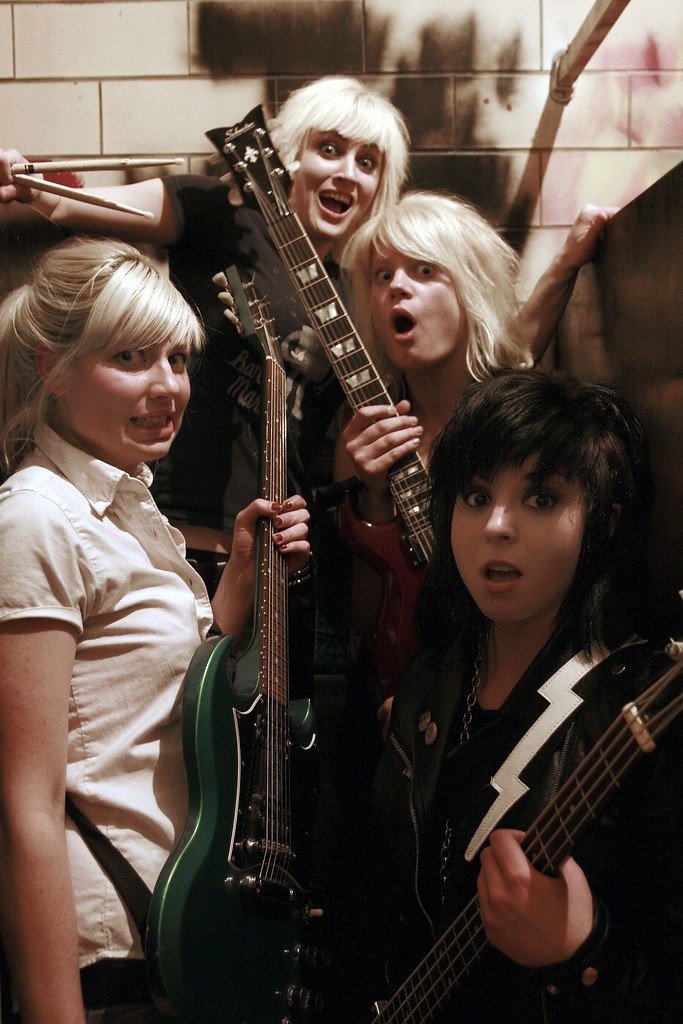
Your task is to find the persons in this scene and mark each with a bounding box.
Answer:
[0,235,311,1024]
[359,370,683,1024]
[0,76,622,695]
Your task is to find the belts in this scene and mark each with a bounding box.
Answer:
[187,561,227,584]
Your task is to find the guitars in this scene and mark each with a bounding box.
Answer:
[140,263,357,1024]
[365,588,682,1023]
[203,102,440,572]
